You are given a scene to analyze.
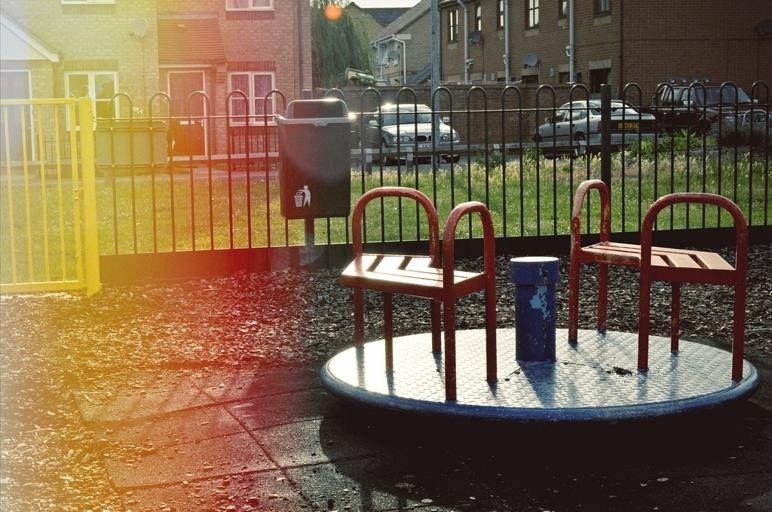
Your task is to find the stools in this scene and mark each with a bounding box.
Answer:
[562,175,750,386]
[332,182,500,405]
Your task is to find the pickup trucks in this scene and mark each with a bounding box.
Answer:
[634,78,771,147]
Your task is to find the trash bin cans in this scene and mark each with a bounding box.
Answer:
[277,97,352,219]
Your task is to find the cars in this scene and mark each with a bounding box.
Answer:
[361,101,463,164]
[529,97,657,158]
